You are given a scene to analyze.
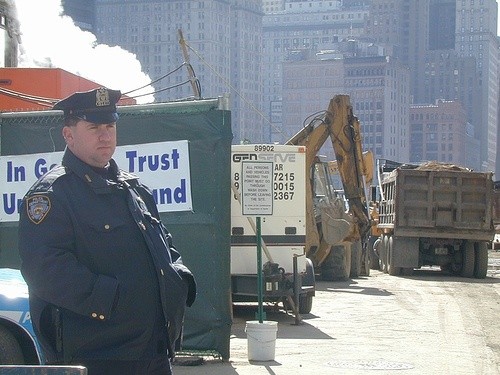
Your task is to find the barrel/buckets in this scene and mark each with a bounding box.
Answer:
[245,320,278,361]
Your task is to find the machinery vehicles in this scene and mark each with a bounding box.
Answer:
[284,94,376,282]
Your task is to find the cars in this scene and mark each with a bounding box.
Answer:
[0,267,42,366]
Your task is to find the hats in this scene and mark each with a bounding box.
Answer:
[51,87,121,124]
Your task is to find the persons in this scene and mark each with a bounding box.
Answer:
[17,87,197,375]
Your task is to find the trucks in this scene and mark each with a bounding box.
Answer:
[366,159,495,278]
